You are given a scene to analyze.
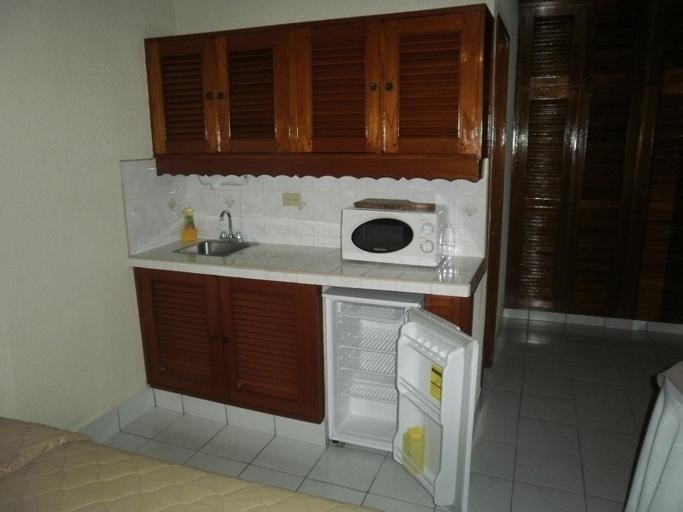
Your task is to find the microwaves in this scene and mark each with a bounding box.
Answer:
[339,207,443,267]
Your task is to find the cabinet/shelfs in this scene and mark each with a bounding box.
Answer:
[134,269,323,424]
[146,29,293,154]
[293,9,482,158]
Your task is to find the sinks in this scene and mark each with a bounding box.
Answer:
[173,241,259,257]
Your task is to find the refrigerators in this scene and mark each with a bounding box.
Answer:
[321,292,482,502]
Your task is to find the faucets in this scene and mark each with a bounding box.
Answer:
[218,209,234,239]
